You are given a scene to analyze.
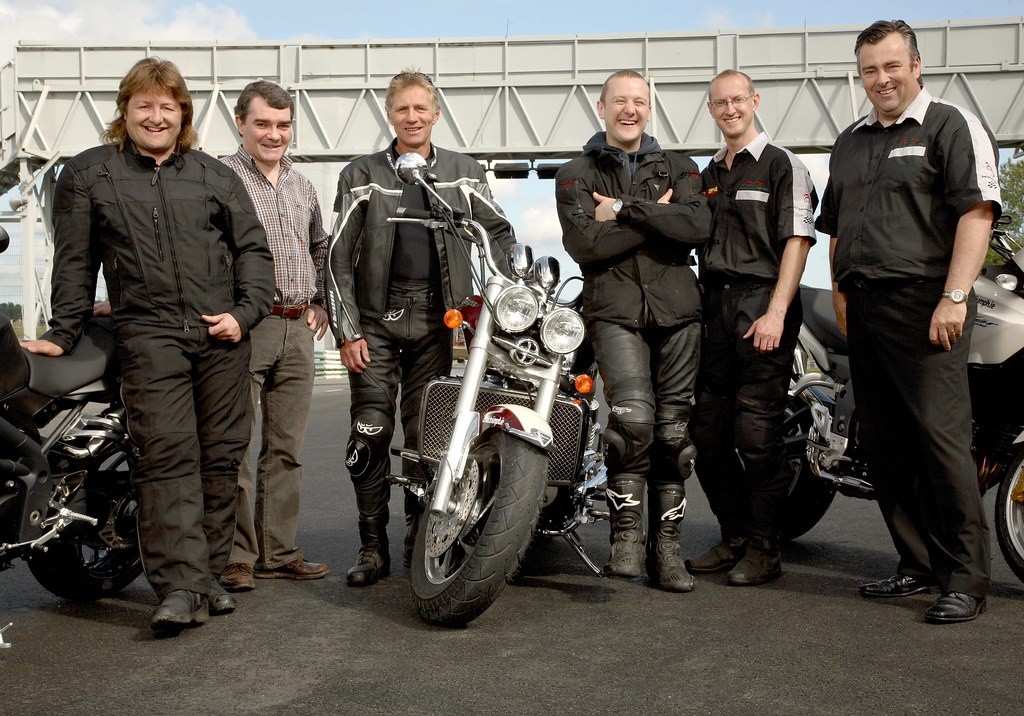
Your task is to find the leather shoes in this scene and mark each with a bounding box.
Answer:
[151,590,208,632]
[858,573,941,596]
[925,591,986,620]
[254,559,328,579]
[219,564,256,590]
[208,583,236,611]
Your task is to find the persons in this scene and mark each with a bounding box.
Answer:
[555,69,712,592]
[686,69,820,587]
[93,80,329,590]
[19,58,276,631]
[814,21,1003,624]
[325,69,517,586]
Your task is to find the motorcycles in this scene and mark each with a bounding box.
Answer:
[777,215,1024,585]
[0,223,145,600]
[384,151,612,630]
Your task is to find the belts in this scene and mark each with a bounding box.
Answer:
[271,305,305,318]
[843,278,946,292]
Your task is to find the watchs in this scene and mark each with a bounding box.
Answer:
[613,198,623,218]
[941,289,969,304]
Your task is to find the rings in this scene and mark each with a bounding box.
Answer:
[767,345,774,346]
[948,330,955,336]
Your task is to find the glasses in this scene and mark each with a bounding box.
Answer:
[390,73,434,87]
[710,93,754,107]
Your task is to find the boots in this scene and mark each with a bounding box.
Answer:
[727,489,782,584]
[346,518,391,587]
[685,503,751,573]
[603,473,647,578]
[646,484,698,591]
[401,516,466,568]
